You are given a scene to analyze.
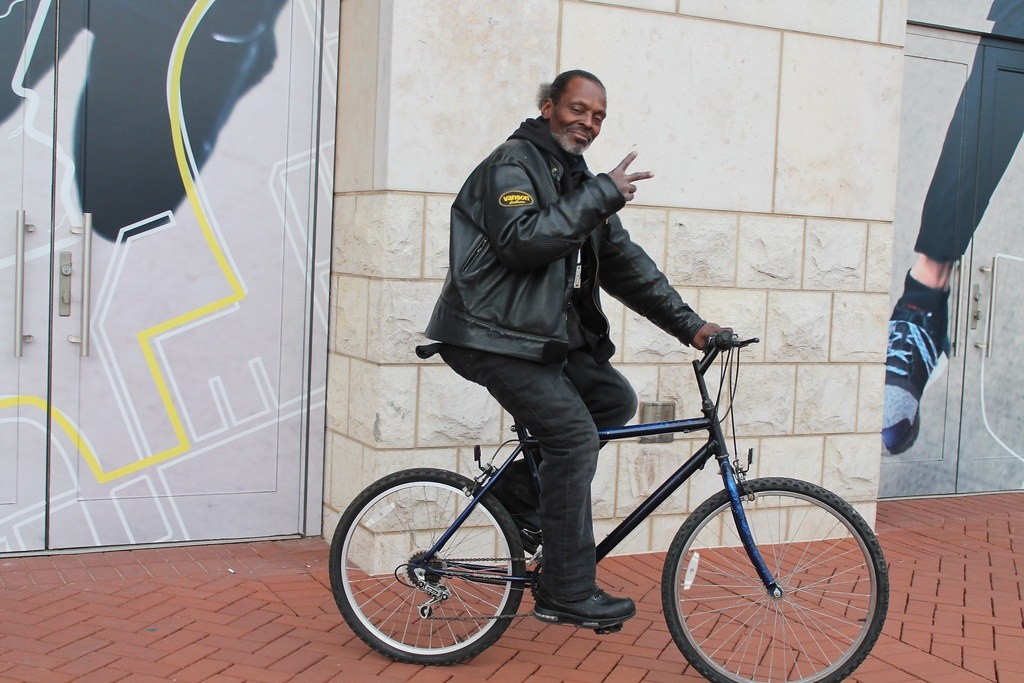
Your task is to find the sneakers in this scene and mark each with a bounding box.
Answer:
[880,301,952,459]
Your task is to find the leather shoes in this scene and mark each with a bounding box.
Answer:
[533,585,638,630]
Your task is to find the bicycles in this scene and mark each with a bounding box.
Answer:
[328,327,889,683]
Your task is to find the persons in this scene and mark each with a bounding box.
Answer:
[0,1,289,242]
[881,0,1024,457]
[425,70,721,629]
